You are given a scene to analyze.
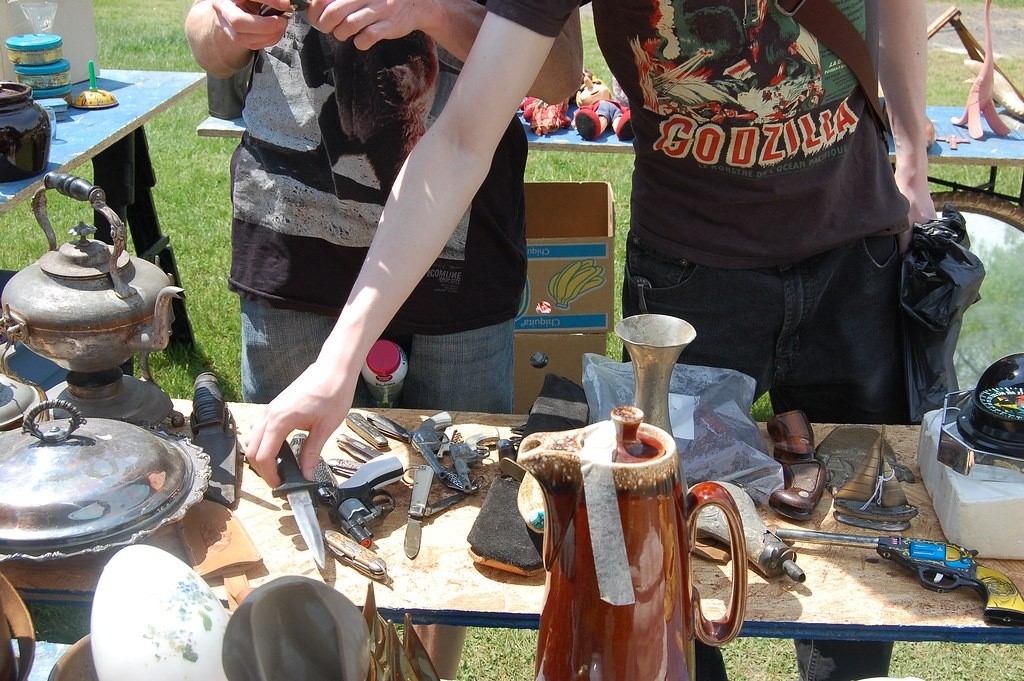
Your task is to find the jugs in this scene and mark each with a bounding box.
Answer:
[514,406,749,681]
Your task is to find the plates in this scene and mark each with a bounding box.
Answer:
[92,546,234,680]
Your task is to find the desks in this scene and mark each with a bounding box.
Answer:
[198,96,1024,214]
[0,68,209,344]
[0,397,1024,643]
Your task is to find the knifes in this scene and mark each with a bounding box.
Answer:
[270,438,327,569]
[402,464,433,559]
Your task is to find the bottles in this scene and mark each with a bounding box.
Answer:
[361,340,405,405]
[6,34,74,108]
[0,81,50,182]
[615,313,698,440]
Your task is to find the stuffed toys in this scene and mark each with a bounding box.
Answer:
[576,77,635,140]
[522,96,571,132]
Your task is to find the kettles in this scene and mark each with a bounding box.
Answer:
[0,170,185,374]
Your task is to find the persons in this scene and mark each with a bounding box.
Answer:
[186,0,585,681]
[246,0,938,681]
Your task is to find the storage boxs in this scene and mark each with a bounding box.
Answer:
[514,332,607,414]
[510,181,616,335]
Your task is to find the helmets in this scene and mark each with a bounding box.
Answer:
[957,353,1024,461]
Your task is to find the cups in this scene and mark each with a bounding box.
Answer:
[20,2,59,37]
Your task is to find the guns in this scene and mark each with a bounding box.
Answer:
[773,528,1024,626]
